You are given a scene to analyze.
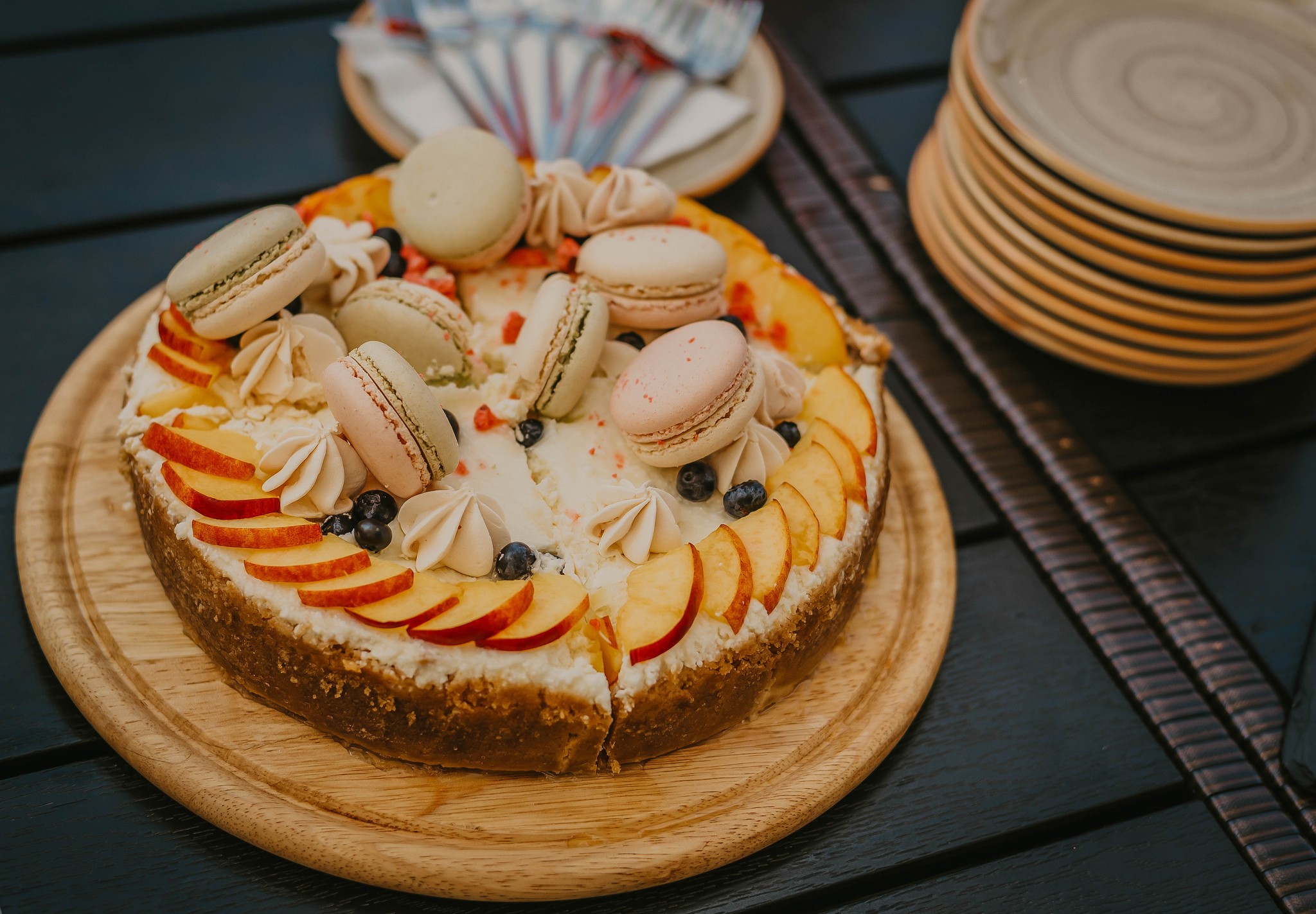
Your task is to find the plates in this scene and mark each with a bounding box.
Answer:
[338,1,783,200]
[908,0,1316,383]
[11,280,956,901]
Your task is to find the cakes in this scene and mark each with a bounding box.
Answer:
[116,126,888,776]
